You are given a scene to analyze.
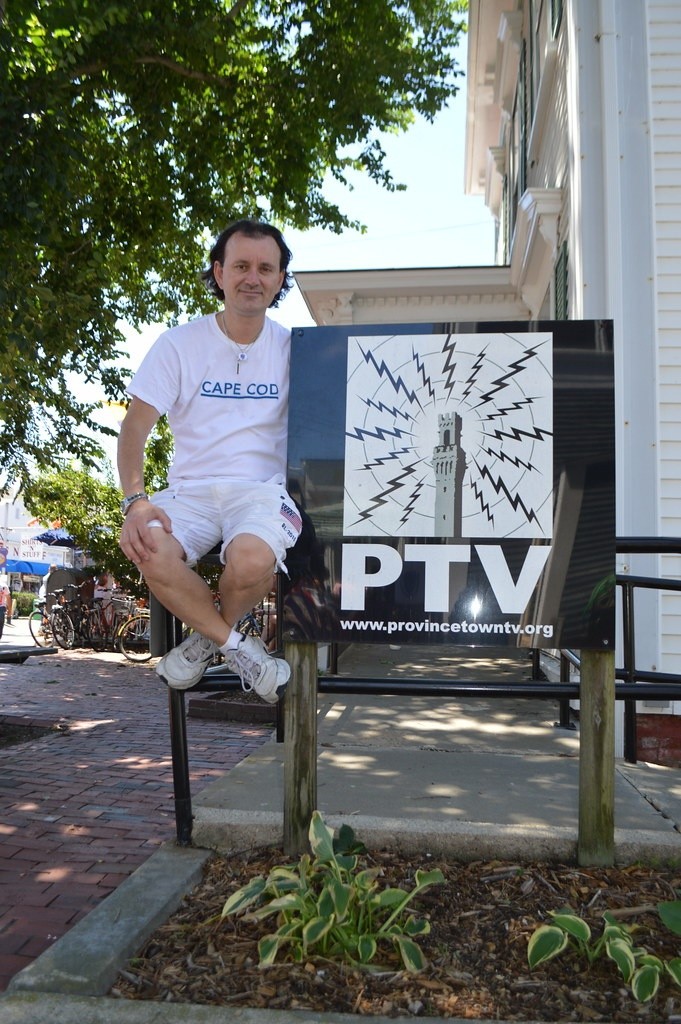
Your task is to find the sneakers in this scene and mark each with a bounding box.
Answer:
[224,632,292,705]
[156,613,221,690]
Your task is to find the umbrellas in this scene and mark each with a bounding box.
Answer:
[30,524,113,550]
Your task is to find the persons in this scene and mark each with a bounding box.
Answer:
[94,577,111,599]
[114,219,303,706]
[0,569,11,639]
[38,564,58,636]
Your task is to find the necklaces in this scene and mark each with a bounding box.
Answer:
[221,311,264,375]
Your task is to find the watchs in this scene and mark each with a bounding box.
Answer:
[120,493,148,516]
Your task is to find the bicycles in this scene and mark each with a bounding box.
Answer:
[28,580,136,652]
[117,598,264,663]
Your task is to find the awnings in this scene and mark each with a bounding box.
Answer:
[5,559,62,575]
